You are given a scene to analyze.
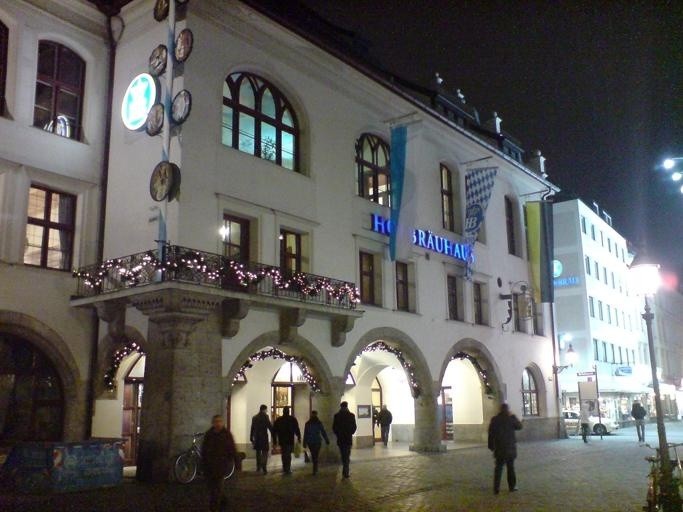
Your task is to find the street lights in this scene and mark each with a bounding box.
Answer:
[620,261,674,493]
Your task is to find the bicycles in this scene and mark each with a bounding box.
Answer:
[172,431,237,485]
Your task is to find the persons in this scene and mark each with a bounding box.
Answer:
[487,402,524,496]
[371,402,378,447]
[377,403,393,446]
[577,402,592,444]
[631,400,647,443]
[271,406,301,476]
[331,400,358,478]
[249,404,274,473]
[302,410,330,475]
[199,413,242,510]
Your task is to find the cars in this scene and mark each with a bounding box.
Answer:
[560,409,615,436]
